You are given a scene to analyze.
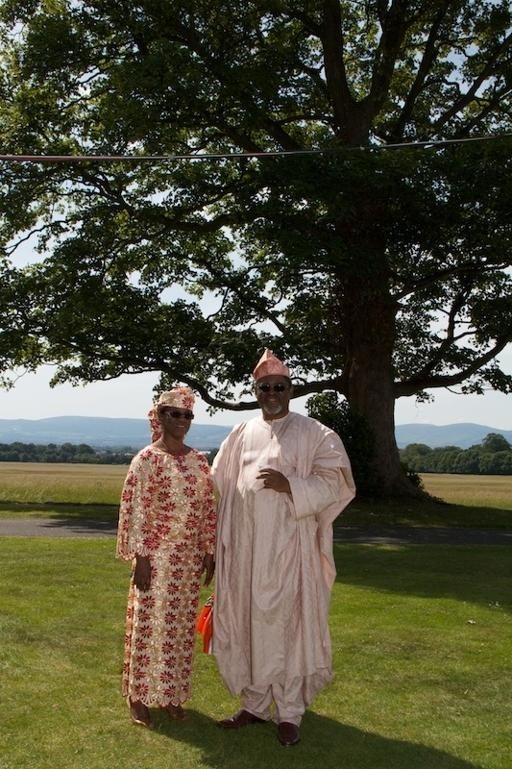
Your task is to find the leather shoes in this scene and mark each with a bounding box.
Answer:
[278,722,300,747]
[126,695,150,728]
[166,702,185,720]
[217,709,262,728]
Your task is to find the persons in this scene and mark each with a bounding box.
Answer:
[208,349,357,747]
[114,386,217,727]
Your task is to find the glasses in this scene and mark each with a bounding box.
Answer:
[165,409,194,420]
[256,383,289,392]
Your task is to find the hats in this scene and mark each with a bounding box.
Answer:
[253,349,290,381]
